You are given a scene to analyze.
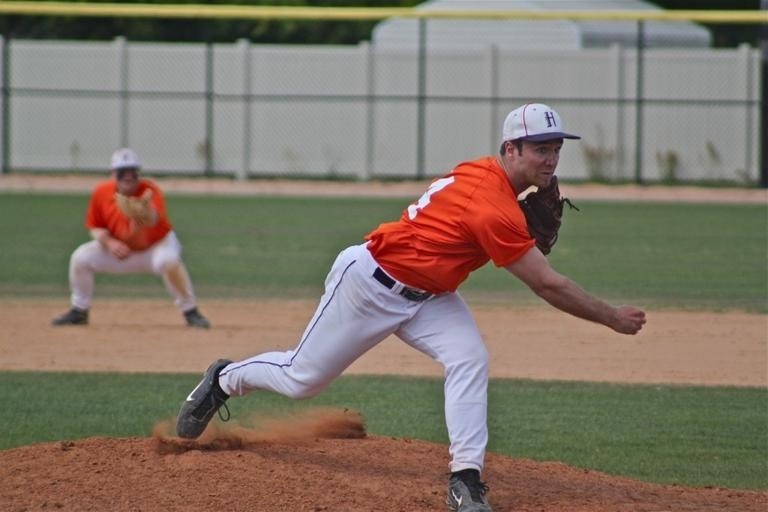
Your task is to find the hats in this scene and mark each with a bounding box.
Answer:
[502,102,580,143]
[110,149,140,173]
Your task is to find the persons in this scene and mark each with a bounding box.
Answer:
[49,148,211,330]
[175,99,647,512]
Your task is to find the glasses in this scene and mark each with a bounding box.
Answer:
[114,171,138,180]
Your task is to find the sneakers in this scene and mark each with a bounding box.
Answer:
[184,308,207,327]
[446,470,491,511]
[51,307,88,324]
[176,360,235,440]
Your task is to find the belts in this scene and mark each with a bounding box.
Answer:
[372,268,432,301]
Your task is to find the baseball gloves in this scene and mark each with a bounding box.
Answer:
[515,175,563,256]
[113,187,154,227]
